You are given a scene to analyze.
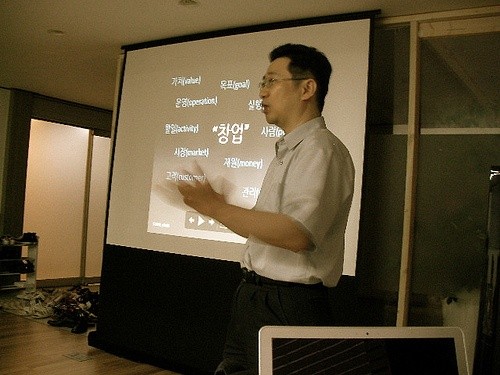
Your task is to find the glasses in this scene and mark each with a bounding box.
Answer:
[259,78,306,88]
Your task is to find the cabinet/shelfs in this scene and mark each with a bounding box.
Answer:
[0,240,37,295]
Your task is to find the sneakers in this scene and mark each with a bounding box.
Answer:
[14,232,38,245]
[0,235,15,245]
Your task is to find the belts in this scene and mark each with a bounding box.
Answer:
[240,268,316,287]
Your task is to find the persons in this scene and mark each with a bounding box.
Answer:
[176,43,354,375]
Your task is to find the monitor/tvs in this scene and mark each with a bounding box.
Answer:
[258,326,470,375]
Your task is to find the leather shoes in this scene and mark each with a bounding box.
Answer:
[47,314,75,327]
[71,317,88,333]
